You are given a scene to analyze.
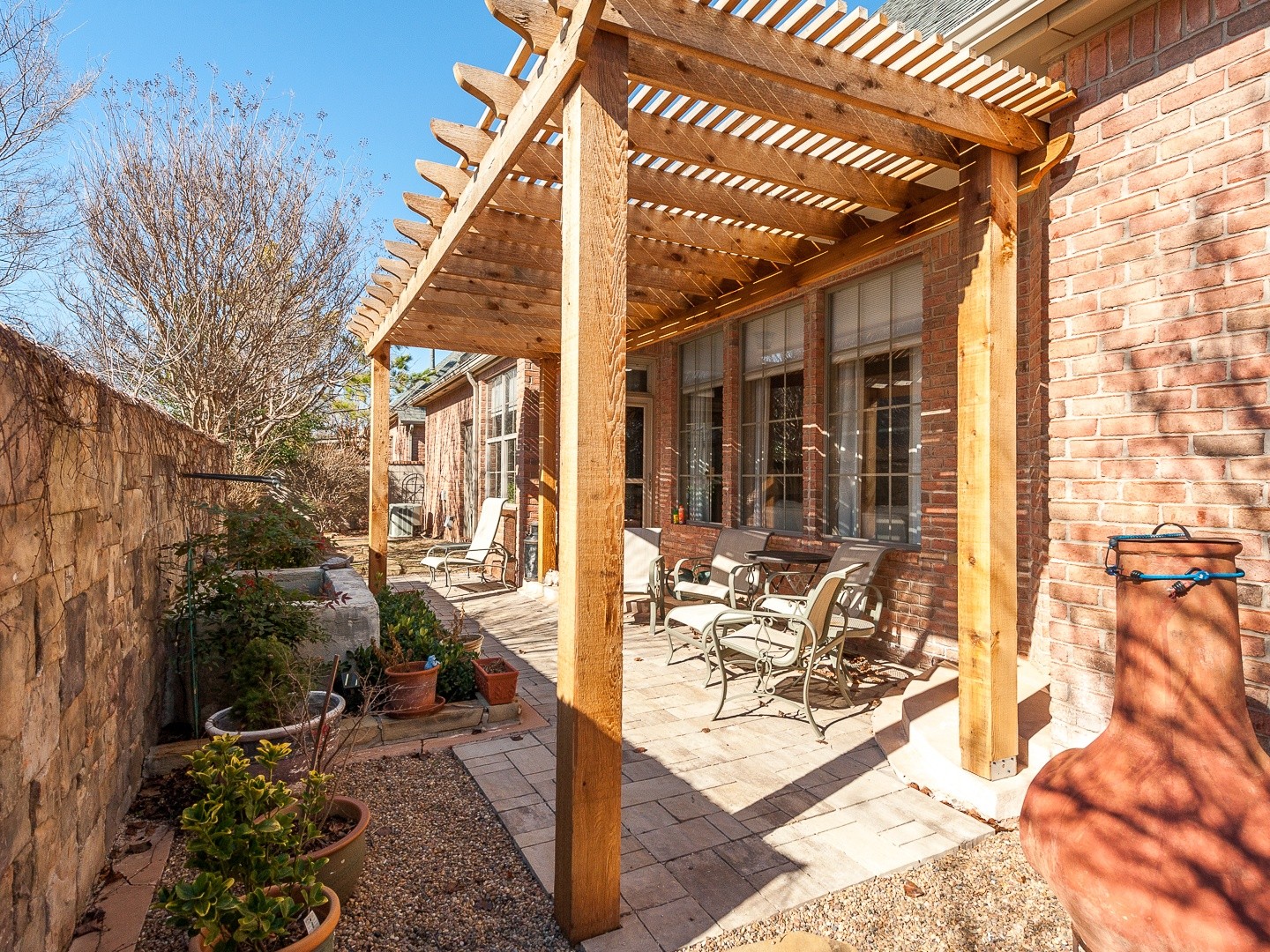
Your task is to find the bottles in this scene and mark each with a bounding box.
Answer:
[672,505,685,524]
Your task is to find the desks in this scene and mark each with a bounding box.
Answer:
[744,550,832,633]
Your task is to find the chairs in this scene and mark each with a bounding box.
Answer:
[420,498,510,598]
[664,527,896,741]
[623,528,666,636]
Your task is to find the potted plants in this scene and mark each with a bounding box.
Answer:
[370,573,520,719]
[151,634,370,952]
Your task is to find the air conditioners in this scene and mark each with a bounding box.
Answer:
[389,503,423,538]
